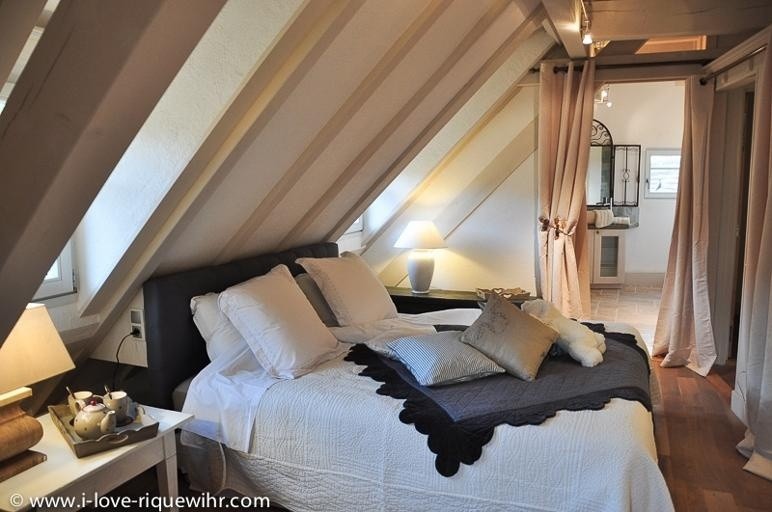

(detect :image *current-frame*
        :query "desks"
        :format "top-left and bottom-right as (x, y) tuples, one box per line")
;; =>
(0, 397), (195, 511)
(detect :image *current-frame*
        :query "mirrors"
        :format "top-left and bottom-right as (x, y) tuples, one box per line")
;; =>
(586, 145), (610, 207)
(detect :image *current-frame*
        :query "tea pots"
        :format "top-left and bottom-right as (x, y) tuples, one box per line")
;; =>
(72, 399), (117, 440)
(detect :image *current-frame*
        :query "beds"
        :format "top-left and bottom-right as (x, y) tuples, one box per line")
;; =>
(144, 242), (660, 510)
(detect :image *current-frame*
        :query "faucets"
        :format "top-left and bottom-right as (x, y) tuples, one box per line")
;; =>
(603, 197), (613, 211)
(596, 197), (607, 207)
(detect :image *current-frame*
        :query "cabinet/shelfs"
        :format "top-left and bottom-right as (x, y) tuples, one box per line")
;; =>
(584, 230), (625, 289)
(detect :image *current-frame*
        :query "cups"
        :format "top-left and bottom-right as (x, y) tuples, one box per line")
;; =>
(66, 391), (92, 420)
(102, 390), (128, 423)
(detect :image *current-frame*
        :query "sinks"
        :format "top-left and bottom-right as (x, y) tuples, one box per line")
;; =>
(586, 208), (610, 224)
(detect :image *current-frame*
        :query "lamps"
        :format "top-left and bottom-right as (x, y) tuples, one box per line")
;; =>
(1, 301), (76, 481)
(393, 221), (447, 294)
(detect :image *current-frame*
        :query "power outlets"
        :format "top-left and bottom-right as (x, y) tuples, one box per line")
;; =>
(129, 310), (145, 341)
